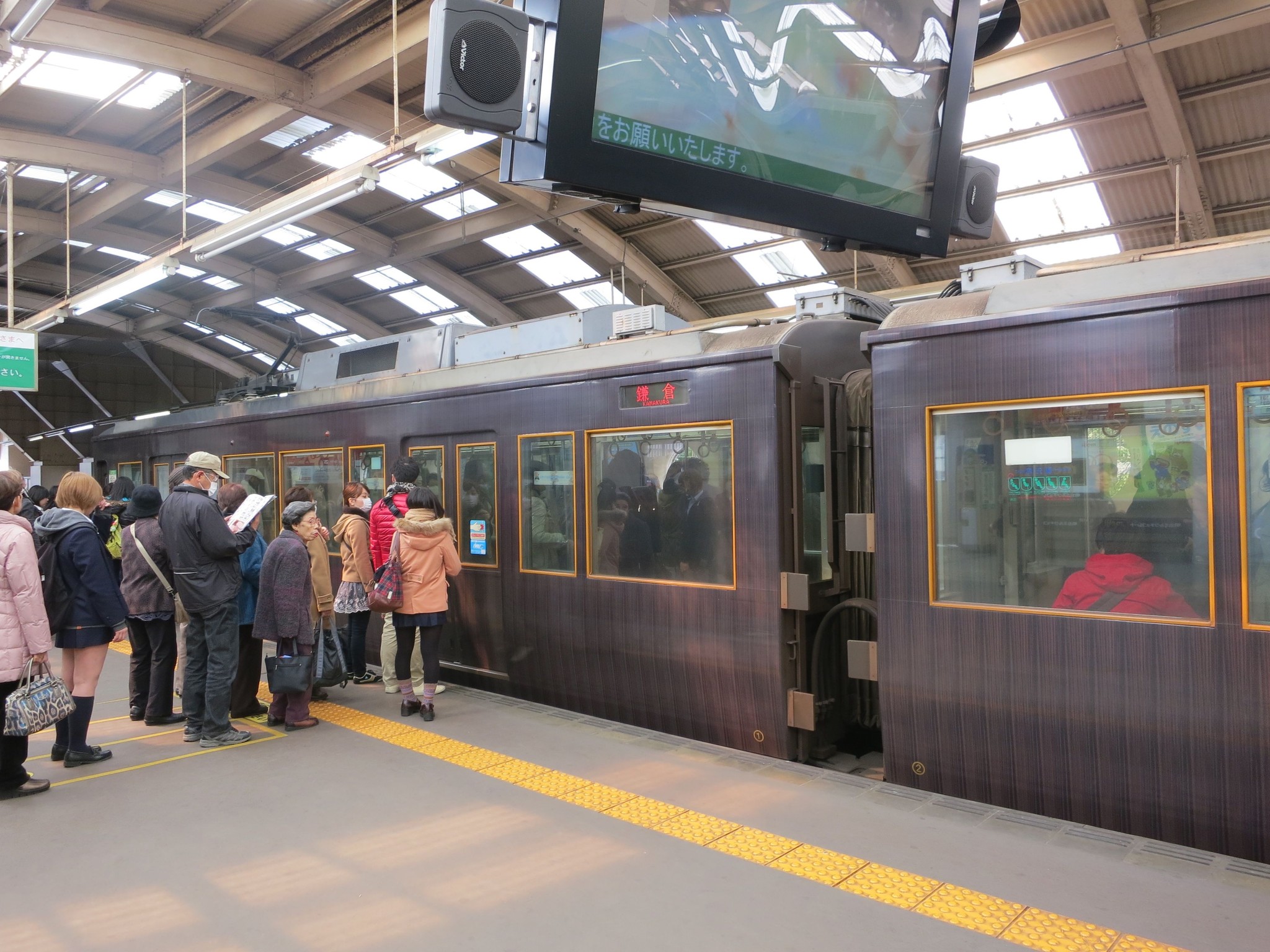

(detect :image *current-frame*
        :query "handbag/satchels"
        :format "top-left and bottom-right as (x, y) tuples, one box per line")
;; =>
(264, 636), (312, 695)
(3, 656), (76, 736)
(170, 591), (193, 627)
(365, 558), (403, 613)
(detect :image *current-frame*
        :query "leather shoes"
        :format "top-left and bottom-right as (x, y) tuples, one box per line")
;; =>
(50, 743), (112, 767)
(129, 705), (188, 726)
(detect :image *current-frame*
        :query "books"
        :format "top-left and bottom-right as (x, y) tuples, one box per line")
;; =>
(227, 494), (277, 533)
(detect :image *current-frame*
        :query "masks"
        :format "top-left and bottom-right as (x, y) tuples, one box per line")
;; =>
(353, 497), (372, 513)
(198, 471), (218, 497)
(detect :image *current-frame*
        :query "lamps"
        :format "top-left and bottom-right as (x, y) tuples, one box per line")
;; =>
(68, 256), (180, 316)
(190, 165), (379, 262)
(0, 0), (56, 45)
(25, 309), (68, 331)
(414, 124), (498, 166)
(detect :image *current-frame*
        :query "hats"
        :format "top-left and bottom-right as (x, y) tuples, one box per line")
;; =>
(126, 484), (163, 517)
(245, 468), (265, 479)
(184, 451), (230, 479)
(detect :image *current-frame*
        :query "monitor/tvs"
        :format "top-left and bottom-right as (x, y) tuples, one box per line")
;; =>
(498, 0), (978, 261)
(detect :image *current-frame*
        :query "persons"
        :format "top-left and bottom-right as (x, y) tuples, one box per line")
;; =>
(463, 449), (733, 583)
(463, 479), (490, 563)
(333, 456), (462, 721)
(0, 465), (52, 797)
(119, 449), (268, 747)
(1051, 512), (1203, 618)
(252, 486), (333, 731)
(90, 476), (135, 587)
(296, 469), (343, 552)
(1127, 442), (1207, 621)
(240, 469), (264, 537)
(1250, 459), (1270, 623)
(18, 485), (59, 551)
(34, 470), (129, 766)
(597, 485), (619, 577)
(414, 464), (443, 505)
(1063, 456), (1115, 583)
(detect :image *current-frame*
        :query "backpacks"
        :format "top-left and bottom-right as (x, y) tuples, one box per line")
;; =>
(105, 514), (123, 559)
(35, 520), (98, 636)
(310, 613), (348, 689)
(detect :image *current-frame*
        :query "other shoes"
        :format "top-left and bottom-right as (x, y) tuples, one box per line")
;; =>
(230, 704), (268, 718)
(0, 778), (51, 801)
(265, 712), (285, 726)
(310, 690), (328, 702)
(284, 717), (319, 732)
(401, 698), (435, 722)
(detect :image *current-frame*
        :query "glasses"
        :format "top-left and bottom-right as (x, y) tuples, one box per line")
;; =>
(300, 517), (320, 527)
(205, 472), (219, 482)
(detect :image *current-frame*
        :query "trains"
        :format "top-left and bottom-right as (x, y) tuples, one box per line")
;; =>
(89, 225), (1270, 864)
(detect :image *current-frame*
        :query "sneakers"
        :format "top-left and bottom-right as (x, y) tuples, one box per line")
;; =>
(200, 724), (252, 747)
(347, 669), (446, 696)
(183, 724), (202, 742)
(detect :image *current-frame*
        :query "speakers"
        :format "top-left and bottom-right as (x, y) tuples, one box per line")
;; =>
(423, 1), (530, 132)
(950, 154), (1000, 239)
